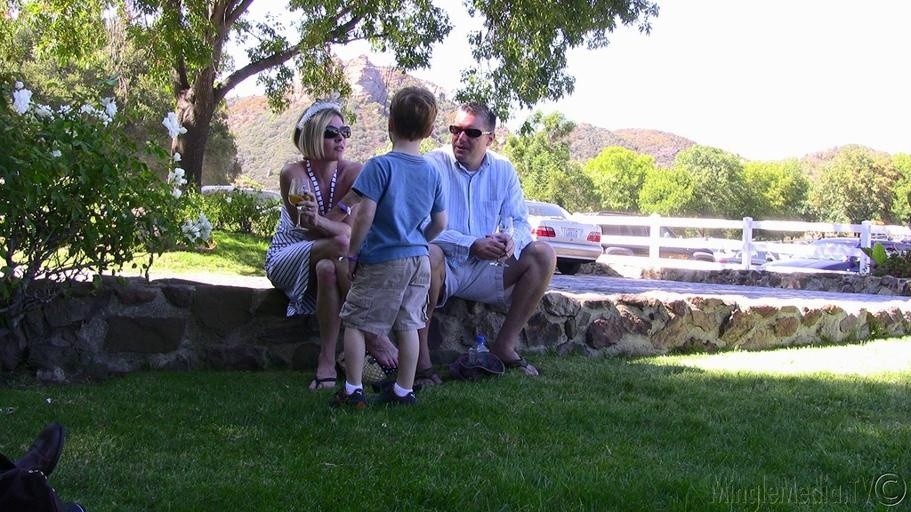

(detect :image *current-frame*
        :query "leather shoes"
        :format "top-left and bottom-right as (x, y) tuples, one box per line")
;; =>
(15, 421), (65, 476)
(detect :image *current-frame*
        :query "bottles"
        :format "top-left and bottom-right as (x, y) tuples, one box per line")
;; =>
(468, 335), (489, 362)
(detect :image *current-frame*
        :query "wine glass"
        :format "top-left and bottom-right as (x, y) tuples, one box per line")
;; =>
(287, 177), (311, 231)
(489, 215), (514, 267)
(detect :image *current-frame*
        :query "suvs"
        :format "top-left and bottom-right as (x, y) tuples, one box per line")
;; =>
(573, 212), (715, 261)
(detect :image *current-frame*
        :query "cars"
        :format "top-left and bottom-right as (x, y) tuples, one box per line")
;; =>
(703, 235), (910, 275)
(525, 204), (604, 275)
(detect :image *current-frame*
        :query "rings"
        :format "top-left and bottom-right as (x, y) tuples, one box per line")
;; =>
(308, 208), (311, 210)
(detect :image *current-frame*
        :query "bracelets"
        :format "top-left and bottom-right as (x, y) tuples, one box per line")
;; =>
(337, 201), (352, 215)
(347, 255), (358, 262)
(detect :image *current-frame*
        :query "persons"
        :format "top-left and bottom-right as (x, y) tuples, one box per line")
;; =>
(329, 86), (448, 408)
(264, 99), (400, 392)
(412, 103), (557, 390)
(0, 422), (86, 512)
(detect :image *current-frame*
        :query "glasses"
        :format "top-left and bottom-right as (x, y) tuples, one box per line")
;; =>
(326, 125), (352, 138)
(450, 125), (492, 138)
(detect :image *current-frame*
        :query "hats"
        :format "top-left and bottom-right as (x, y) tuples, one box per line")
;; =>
(448, 350), (506, 381)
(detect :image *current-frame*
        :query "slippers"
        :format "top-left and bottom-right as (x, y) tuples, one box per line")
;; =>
(503, 354), (530, 369)
(415, 367), (437, 380)
(310, 374), (338, 391)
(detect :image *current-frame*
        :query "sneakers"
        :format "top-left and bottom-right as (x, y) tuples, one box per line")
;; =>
(379, 383), (416, 405)
(332, 386), (368, 409)
